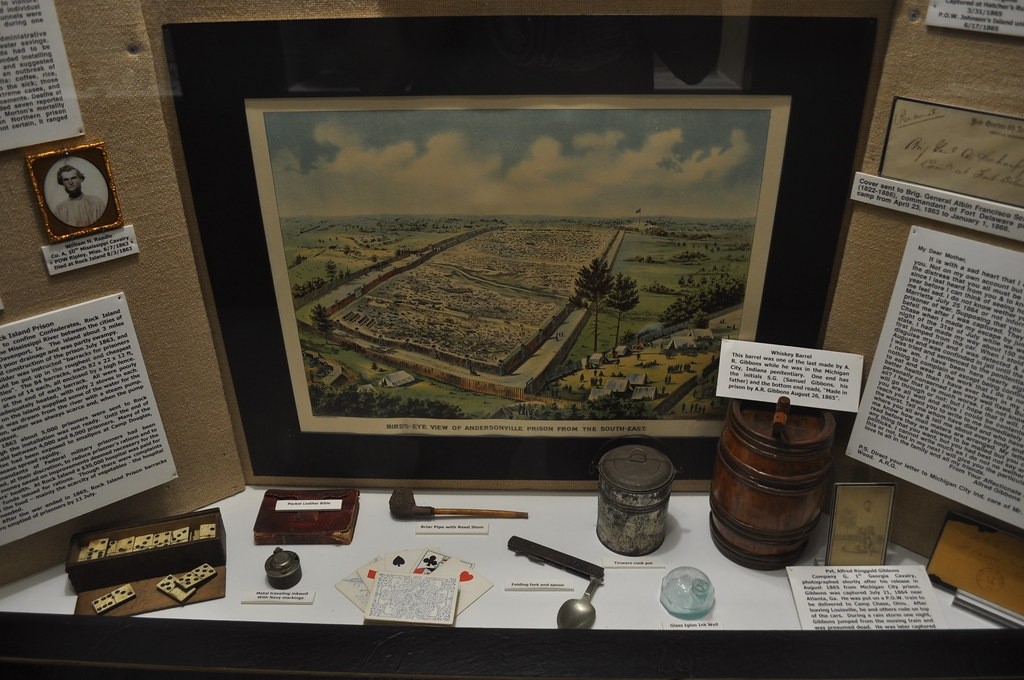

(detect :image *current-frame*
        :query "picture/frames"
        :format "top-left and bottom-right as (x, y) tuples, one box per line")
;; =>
(26, 140), (123, 242)
(824, 482), (897, 566)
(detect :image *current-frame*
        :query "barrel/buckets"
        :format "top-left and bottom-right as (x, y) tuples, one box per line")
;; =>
(593, 434), (676, 556)
(710, 397), (834, 570)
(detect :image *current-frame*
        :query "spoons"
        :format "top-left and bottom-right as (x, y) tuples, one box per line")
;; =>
(507, 536), (605, 627)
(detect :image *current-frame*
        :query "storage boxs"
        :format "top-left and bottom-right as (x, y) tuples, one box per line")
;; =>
(63, 507), (226, 592)
